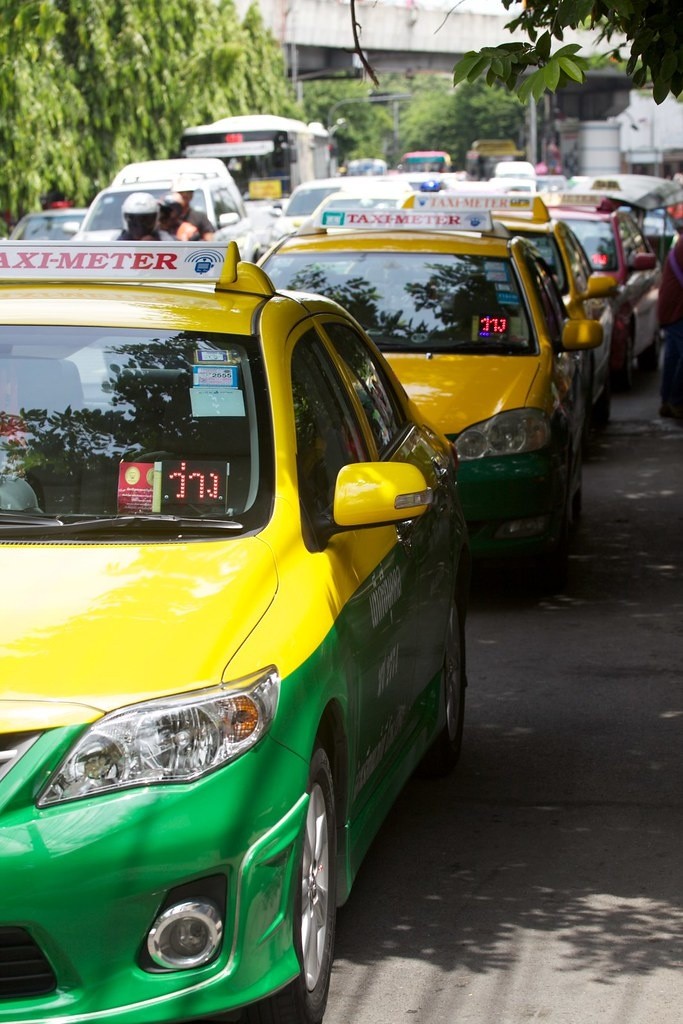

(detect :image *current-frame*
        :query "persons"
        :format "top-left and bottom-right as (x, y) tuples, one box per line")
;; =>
(119, 186), (215, 242)
(656, 222), (683, 424)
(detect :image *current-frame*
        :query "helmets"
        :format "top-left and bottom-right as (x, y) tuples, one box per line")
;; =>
(121, 192), (159, 232)
(157, 192), (186, 229)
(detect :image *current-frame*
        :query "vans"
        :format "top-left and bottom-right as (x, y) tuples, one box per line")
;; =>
(61, 157), (246, 247)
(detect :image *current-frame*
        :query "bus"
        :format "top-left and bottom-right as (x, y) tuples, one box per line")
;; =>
(176, 113), (333, 206)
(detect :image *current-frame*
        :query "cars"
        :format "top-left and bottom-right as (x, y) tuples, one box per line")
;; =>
(0, 239), (469, 1024)
(0, 208), (87, 242)
(212, 137), (683, 458)
(250, 206), (603, 601)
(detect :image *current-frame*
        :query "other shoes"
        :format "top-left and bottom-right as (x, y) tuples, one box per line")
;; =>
(659, 404), (683, 418)
